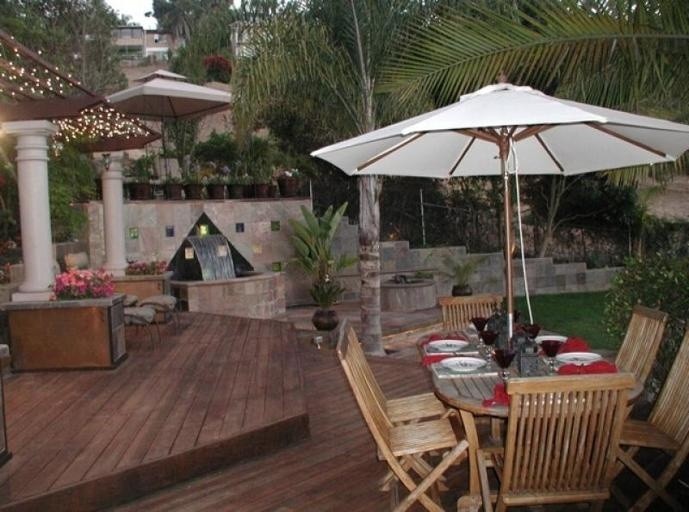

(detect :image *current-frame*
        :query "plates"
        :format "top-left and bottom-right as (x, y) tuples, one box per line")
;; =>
(535, 335), (602, 366)
(429, 339), (488, 372)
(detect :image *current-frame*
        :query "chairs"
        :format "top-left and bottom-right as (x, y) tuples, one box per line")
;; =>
(456, 371), (638, 511)
(611, 327), (689, 511)
(64, 250), (183, 333)
(437, 295), (505, 330)
(614, 303), (669, 421)
(336, 316), (457, 512)
(53, 257), (162, 346)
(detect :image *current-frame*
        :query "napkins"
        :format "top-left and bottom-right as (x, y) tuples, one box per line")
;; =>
(421, 354), (458, 367)
(418, 333), (466, 348)
(539, 334), (594, 354)
(482, 381), (513, 408)
(557, 360), (618, 375)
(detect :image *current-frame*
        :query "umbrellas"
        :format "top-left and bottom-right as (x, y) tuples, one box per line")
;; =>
(310, 72), (689, 353)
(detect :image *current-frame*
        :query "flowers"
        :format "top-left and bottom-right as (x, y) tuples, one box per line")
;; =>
(52, 267), (113, 299)
(125, 255), (166, 274)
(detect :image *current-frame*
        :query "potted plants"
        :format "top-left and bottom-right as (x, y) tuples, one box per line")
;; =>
(123, 155), (299, 201)
(419, 253), (492, 297)
(281, 201), (361, 330)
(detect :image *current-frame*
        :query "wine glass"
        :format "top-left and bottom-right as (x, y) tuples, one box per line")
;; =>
(470, 309), (564, 380)
